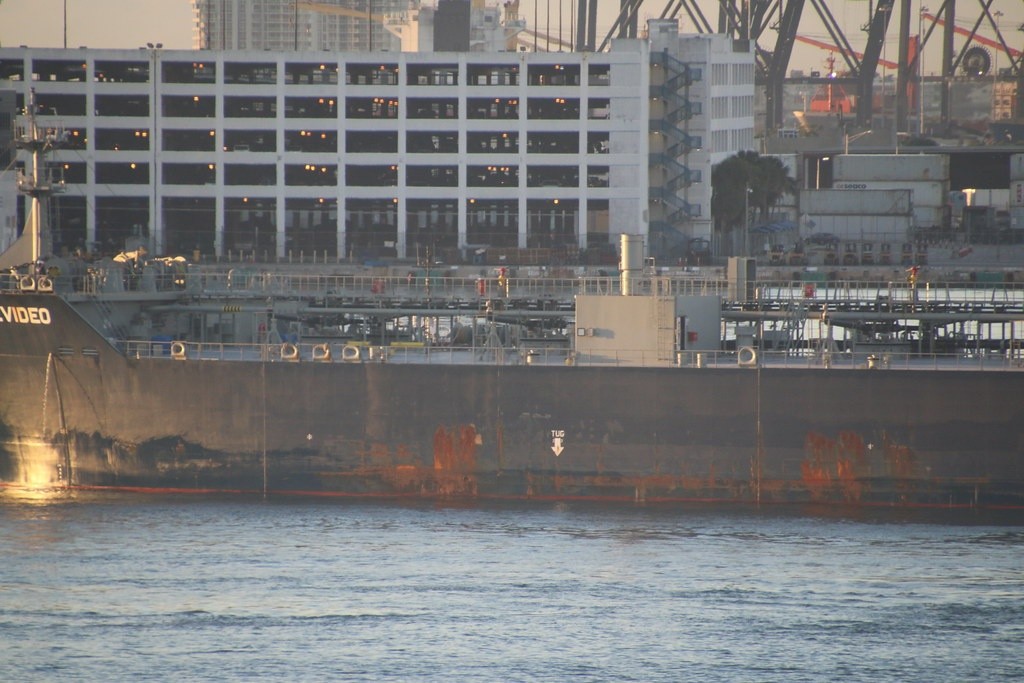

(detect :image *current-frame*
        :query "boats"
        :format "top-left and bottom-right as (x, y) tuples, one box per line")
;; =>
(0, 86), (1024, 522)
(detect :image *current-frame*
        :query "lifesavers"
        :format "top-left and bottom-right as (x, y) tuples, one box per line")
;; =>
(341, 345), (360, 360)
(38, 277), (55, 290)
(738, 346), (757, 365)
(20, 276), (36, 290)
(170, 342), (185, 357)
(312, 344), (331, 360)
(281, 345), (298, 359)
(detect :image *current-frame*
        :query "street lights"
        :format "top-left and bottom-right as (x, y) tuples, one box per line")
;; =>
(992, 10), (1004, 128)
(878, 5), (890, 133)
(919, 7), (931, 134)
(816, 154), (829, 190)
(846, 130), (873, 154)
(744, 186), (753, 259)
